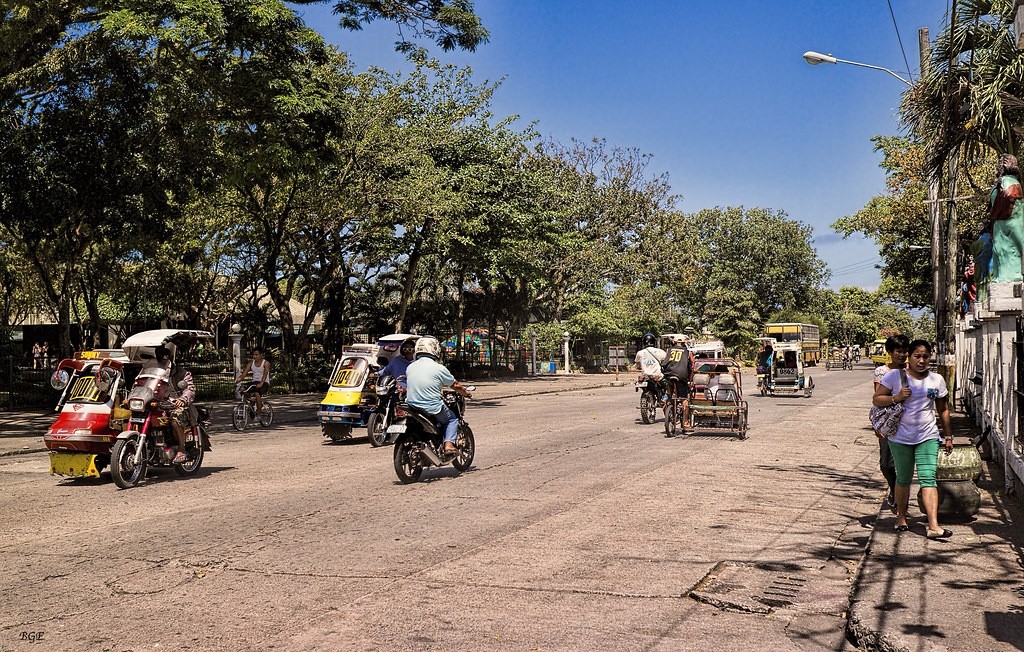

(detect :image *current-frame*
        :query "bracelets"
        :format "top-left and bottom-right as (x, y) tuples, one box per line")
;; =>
(892, 394), (896, 404)
(944, 435), (954, 440)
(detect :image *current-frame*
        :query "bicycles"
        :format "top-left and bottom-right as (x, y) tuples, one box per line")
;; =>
(232, 383), (274, 432)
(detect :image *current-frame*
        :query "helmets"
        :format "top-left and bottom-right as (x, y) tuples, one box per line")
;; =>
(415, 335), (441, 360)
(643, 334), (656, 346)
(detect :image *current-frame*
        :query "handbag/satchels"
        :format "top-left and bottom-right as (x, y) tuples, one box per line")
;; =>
(869, 369), (909, 437)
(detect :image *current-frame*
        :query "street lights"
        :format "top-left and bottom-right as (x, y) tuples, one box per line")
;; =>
(802, 52), (947, 378)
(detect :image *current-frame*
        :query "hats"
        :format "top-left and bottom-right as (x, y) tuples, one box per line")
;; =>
(674, 334), (685, 342)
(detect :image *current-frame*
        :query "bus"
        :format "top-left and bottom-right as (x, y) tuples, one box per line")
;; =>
(763, 323), (821, 367)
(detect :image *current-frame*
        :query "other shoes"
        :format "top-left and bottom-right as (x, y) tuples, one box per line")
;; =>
(253, 409), (261, 423)
(444, 449), (459, 455)
(888, 491), (896, 506)
(894, 517), (909, 531)
(927, 528), (953, 538)
(173, 453), (192, 462)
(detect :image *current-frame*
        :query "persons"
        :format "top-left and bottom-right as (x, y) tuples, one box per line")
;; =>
(690, 352), (708, 392)
(32, 342), (50, 369)
(370, 340), (415, 389)
(758, 345), (773, 368)
(155, 348), (195, 462)
(661, 334), (693, 427)
(635, 333), (666, 387)
(236, 348), (270, 422)
(845, 347), (859, 356)
(405, 335), (471, 454)
(874, 335), (910, 517)
(872, 340), (953, 539)
(704, 365), (737, 401)
(955, 153), (1024, 317)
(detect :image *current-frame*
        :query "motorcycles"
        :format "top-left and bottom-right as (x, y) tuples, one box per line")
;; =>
(386, 385), (476, 484)
(634, 375), (681, 425)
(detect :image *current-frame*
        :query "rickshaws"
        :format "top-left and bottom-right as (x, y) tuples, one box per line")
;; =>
(663, 357), (749, 440)
(755, 337), (815, 398)
(317, 333), (423, 448)
(825, 353), (853, 371)
(43, 329), (214, 488)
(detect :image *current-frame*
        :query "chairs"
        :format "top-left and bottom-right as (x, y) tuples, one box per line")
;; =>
(688, 387), (714, 426)
(715, 389), (741, 427)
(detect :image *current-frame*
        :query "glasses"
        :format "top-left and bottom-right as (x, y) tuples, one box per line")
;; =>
(403, 348), (415, 352)
